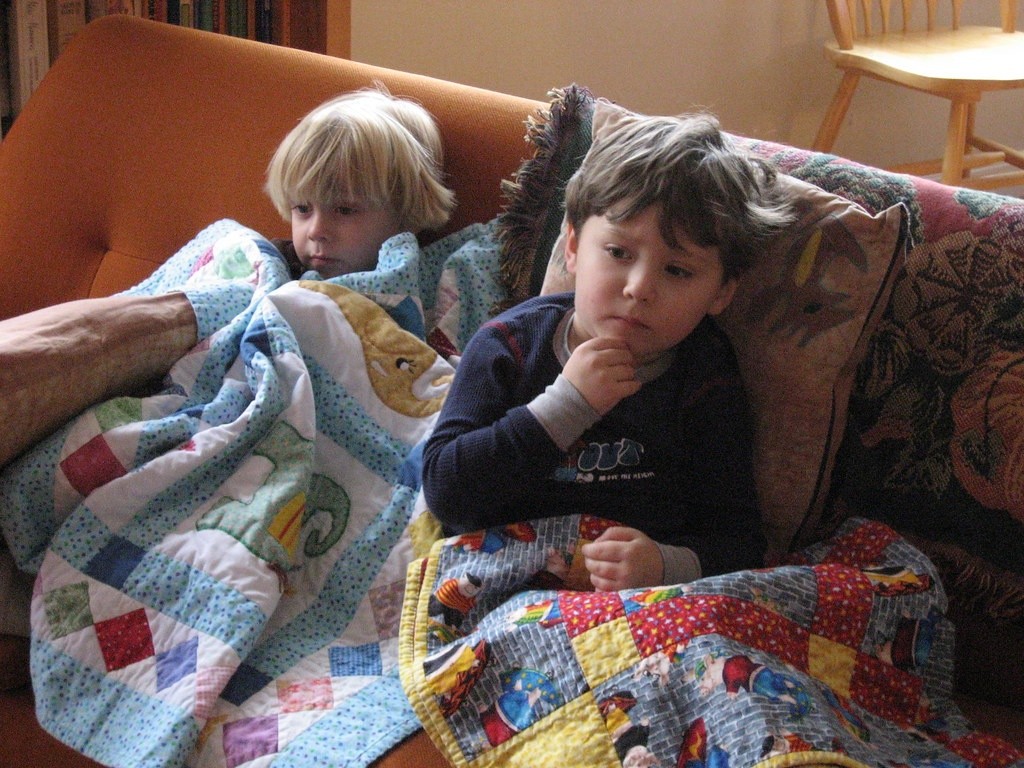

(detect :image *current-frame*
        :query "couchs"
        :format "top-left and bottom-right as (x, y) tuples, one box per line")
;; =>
(1, 11), (1024, 768)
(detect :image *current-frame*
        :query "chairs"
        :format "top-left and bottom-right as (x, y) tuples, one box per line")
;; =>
(810, 0), (1024, 190)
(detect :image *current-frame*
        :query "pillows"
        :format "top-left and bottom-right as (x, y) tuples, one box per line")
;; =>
(0, 290), (198, 469)
(541, 95), (910, 552)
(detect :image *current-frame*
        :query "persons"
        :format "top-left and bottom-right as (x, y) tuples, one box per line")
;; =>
(261, 86), (458, 283)
(420, 112), (768, 591)
